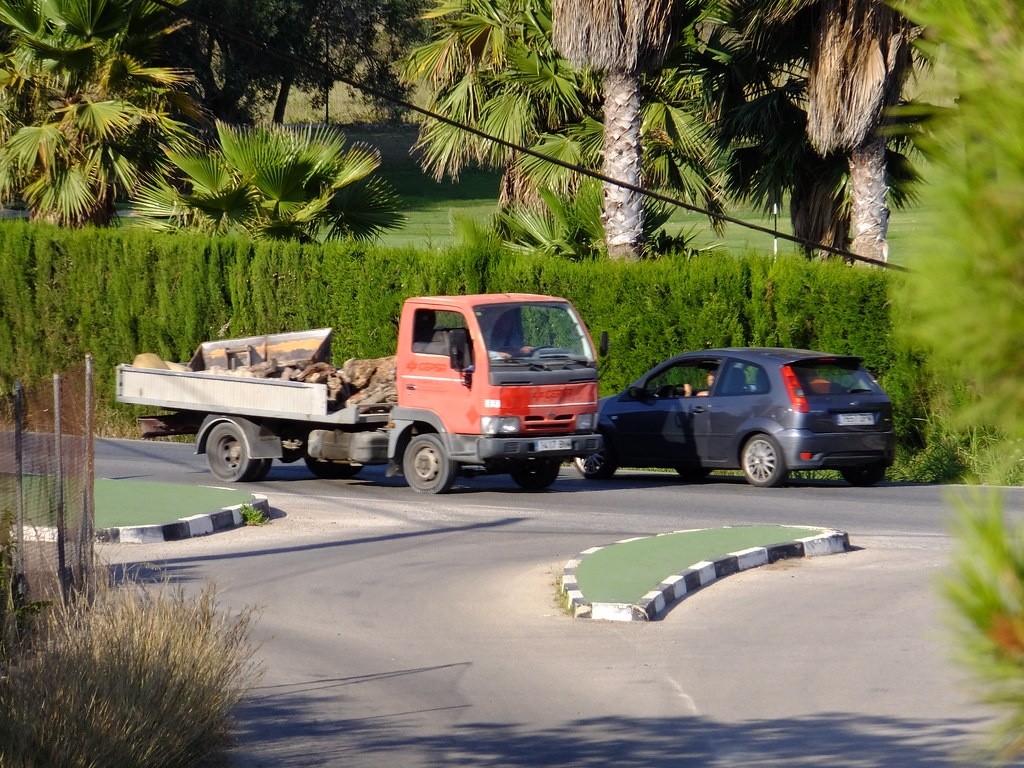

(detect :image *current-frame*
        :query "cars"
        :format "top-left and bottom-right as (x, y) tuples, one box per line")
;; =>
(572, 345), (897, 487)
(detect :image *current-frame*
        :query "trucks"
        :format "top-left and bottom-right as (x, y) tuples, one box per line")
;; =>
(114, 292), (610, 495)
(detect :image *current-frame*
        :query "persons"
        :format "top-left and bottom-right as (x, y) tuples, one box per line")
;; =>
(683, 372), (716, 397)
(484, 308), (534, 355)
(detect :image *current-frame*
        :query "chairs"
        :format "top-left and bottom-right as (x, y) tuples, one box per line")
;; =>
(440, 329), (470, 370)
